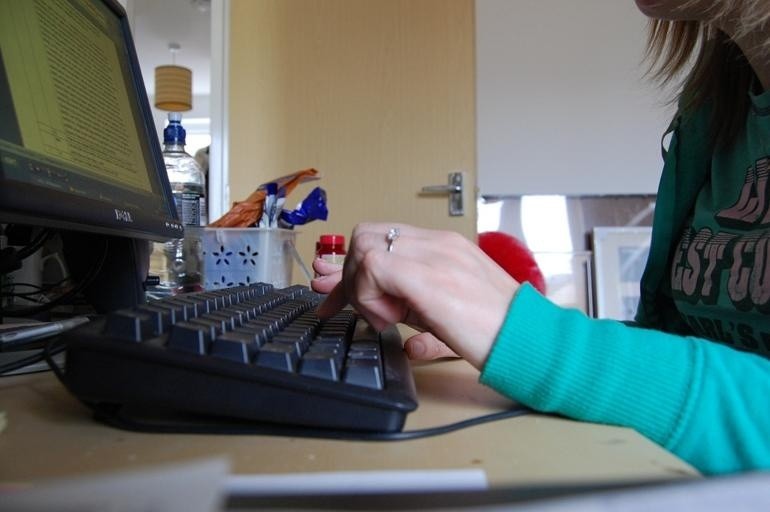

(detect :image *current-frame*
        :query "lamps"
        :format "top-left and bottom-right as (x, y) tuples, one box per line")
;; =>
(153, 42), (194, 112)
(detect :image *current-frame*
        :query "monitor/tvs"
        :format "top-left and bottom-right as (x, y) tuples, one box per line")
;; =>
(0, 0), (185, 344)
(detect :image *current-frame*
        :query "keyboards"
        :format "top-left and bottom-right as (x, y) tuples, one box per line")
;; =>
(62, 279), (419, 438)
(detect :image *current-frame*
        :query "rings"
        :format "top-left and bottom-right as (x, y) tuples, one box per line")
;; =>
(387, 228), (400, 252)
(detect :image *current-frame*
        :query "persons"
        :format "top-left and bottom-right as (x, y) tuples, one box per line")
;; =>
(311, 0), (770, 475)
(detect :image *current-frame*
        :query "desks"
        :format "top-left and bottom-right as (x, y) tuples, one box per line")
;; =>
(1, 327), (702, 484)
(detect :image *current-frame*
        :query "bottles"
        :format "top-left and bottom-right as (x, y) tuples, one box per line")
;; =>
(143, 110), (206, 303)
(317, 235), (347, 268)
